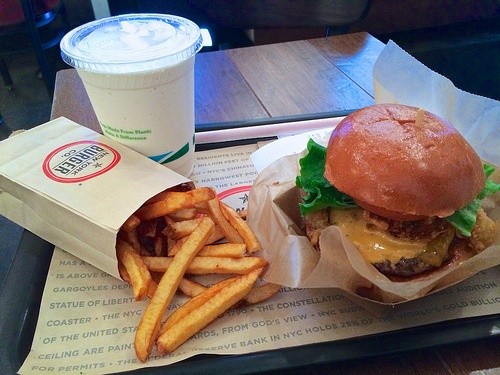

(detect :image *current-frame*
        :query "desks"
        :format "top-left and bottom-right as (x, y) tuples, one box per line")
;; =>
(49, 30), (500, 374)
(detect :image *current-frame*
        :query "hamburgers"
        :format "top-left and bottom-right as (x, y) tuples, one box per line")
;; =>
(273, 103), (500, 299)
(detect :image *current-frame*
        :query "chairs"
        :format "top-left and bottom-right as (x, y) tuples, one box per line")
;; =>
(200, 0), (372, 45)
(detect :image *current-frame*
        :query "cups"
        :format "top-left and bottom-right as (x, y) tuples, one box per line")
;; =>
(58, 13), (205, 179)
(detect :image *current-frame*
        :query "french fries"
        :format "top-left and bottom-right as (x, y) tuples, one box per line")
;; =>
(117, 186), (281, 364)
